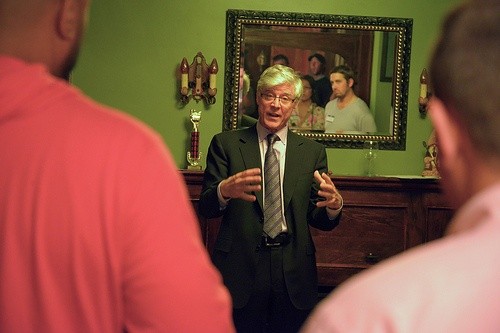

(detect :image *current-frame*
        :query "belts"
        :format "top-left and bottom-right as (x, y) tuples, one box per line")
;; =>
(258, 237), (287, 248)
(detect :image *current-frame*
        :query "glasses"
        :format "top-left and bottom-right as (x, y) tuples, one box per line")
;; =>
(260, 93), (297, 105)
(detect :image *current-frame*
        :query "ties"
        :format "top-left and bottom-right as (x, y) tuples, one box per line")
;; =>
(264, 133), (284, 238)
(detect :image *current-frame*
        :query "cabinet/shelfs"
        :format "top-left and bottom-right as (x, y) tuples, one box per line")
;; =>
(179, 170), (455, 300)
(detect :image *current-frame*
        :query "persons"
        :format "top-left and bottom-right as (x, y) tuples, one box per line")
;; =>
(0, 0), (234, 333)
(299, 0), (500, 333)
(238, 53), (378, 136)
(198, 64), (344, 333)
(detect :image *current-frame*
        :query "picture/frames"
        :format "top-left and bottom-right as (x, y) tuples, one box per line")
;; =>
(222, 9), (414, 151)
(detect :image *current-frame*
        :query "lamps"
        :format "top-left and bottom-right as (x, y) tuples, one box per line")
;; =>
(419, 69), (430, 114)
(180, 51), (218, 105)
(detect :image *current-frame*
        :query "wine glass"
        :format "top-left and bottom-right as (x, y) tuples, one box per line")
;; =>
(361, 140), (378, 176)
